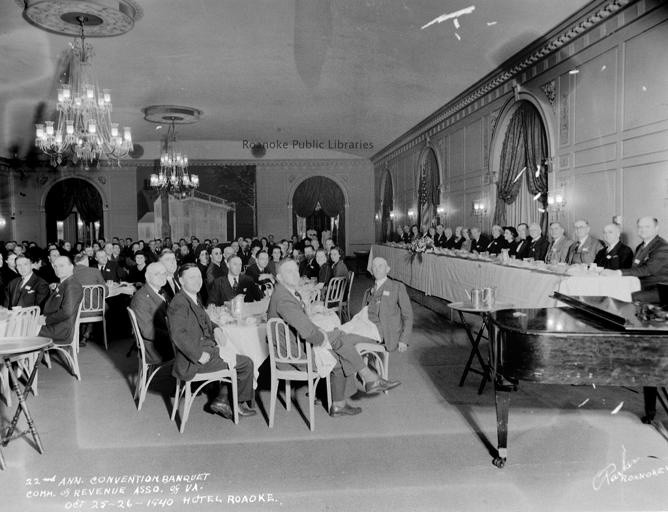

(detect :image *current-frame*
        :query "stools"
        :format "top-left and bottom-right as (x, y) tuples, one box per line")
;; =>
(353, 344), (390, 389)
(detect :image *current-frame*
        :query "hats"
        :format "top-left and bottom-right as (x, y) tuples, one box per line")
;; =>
(195, 243), (212, 258)
(502, 226), (518, 237)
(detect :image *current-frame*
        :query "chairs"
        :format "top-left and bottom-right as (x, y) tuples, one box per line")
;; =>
(321, 276), (347, 318)
(342, 271), (354, 319)
(1, 278), (137, 401)
(160, 315), (236, 432)
(265, 316), (332, 430)
(126, 308), (165, 409)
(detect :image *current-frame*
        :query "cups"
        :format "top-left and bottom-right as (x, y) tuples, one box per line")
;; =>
(208, 276), (318, 326)
(484, 289), (495, 306)
(387, 241), (605, 277)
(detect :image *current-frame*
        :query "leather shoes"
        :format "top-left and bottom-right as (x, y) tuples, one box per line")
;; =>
(210, 399), (233, 418)
(238, 401), (256, 416)
(366, 376), (401, 394)
(331, 403), (362, 417)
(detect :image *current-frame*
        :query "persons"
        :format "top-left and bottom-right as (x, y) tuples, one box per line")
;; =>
(0, 215), (668, 420)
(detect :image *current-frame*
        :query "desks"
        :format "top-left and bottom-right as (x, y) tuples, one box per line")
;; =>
(366, 241), (637, 336)
(1, 337), (53, 467)
(446, 299), (512, 395)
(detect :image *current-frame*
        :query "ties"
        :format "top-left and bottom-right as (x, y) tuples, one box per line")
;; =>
(233, 278), (238, 289)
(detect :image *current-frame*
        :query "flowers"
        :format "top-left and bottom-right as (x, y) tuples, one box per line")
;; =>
(405, 232), (436, 264)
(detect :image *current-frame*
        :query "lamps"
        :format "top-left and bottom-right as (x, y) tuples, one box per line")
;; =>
(137, 103), (206, 201)
(370, 192), (568, 221)
(24, 0), (141, 174)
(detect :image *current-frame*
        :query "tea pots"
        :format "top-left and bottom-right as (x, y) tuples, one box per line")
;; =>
(463, 288), (491, 307)
